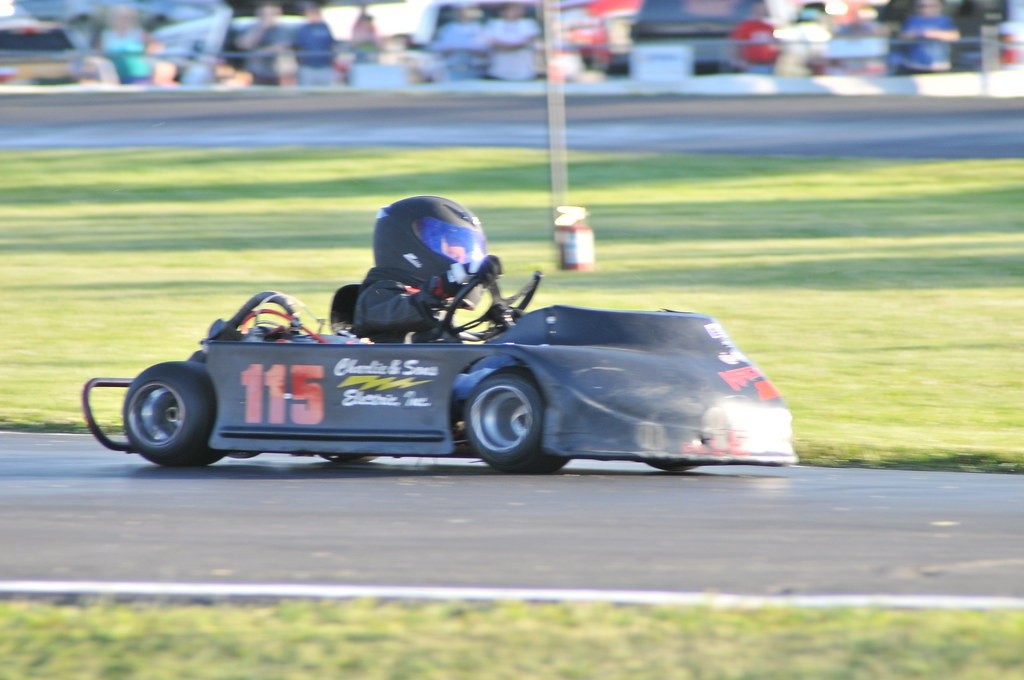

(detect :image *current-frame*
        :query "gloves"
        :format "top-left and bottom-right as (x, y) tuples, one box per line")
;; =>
(437, 256), (503, 293)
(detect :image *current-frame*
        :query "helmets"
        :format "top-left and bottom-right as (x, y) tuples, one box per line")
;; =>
(374, 196), (485, 297)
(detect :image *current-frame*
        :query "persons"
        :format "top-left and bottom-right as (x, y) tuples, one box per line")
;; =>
(431, 3), (484, 82)
(213, 0), (382, 90)
(734, 1), (961, 75)
(485, 3), (541, 80)
(351, 195), (504, 345)
(96, 3), (178, 85)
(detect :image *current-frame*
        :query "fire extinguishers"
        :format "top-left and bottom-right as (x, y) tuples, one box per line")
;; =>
(552, 205), (595, 270)
